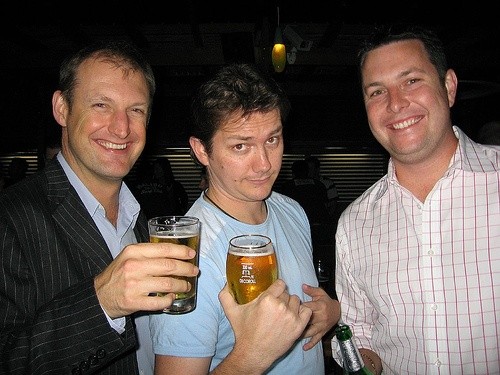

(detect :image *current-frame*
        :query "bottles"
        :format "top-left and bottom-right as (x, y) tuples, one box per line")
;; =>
(334, 324), (376, 375)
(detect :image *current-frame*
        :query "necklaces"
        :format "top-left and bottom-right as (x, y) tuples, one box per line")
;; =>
(203, 187), (268, 224)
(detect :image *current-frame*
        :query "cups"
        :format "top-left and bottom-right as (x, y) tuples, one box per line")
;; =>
(225, 234), (279, 305)
(147, 216), (201, 315)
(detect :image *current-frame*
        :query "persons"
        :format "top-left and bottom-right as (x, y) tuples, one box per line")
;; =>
(6, 157), (28, 189)
(0, 39), (199, 375)
(331, 25), (500, 375)
(149, 63), (341, 375)
(281, 153), (339, 223)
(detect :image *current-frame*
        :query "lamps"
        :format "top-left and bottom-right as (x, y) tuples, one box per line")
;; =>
(272, 6), (286, 74)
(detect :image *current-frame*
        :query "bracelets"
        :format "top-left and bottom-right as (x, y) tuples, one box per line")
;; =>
(360, 353), (378, 374)
